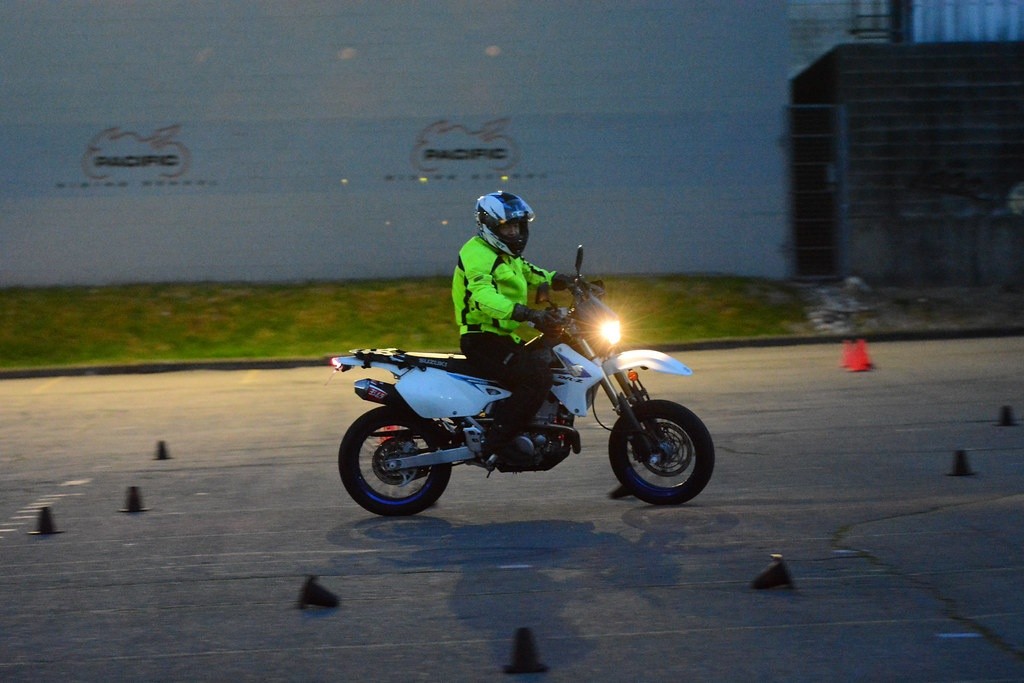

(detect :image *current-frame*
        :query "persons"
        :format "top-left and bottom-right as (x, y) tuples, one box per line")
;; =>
(451, 191), (605, 464)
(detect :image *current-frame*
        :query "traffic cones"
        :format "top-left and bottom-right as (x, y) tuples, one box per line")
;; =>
(837, 336), (873, 373)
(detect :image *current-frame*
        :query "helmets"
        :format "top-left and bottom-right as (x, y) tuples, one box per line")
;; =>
(475, 191), (535, 258)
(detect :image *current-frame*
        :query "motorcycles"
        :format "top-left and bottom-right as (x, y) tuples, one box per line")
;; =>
(329, 242), (717, 519)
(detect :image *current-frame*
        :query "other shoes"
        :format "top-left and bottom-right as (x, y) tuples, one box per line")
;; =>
(480, 432), (530, 468)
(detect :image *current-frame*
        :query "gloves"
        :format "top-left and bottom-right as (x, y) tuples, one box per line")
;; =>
(528, 308), (557, 326)
(553, 273), (581, 295)
